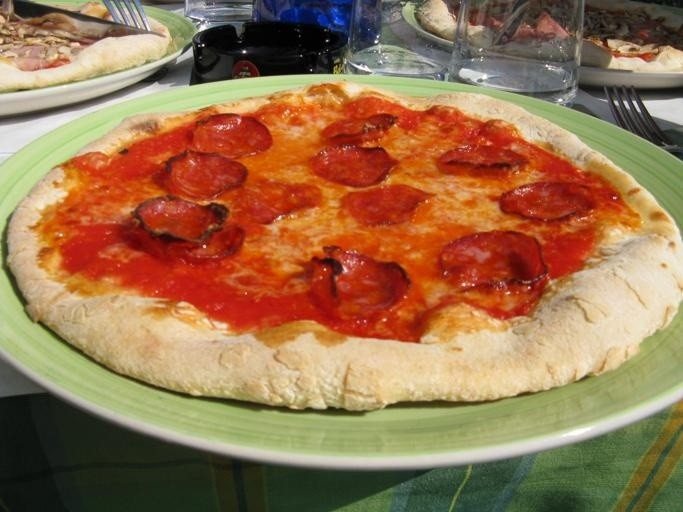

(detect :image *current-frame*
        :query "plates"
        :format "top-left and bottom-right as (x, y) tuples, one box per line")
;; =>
(1, 1), (196, 116)
(3, 72), (683, 473)
(395, 1), (683, 93)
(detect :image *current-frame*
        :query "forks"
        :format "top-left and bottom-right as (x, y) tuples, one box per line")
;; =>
(104, 1), (151, 31)
(604, 83), (683, 159)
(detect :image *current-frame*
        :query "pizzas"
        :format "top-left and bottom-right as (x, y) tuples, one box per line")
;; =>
(6, 83), (683, 410)
(1, 0), (172, 96)
(414, 0), (682, 77)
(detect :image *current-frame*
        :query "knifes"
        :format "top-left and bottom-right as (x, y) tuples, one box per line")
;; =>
(0, 0), (168, 40)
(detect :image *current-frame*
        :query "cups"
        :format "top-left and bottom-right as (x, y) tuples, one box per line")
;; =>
(344, 1), (462, 78)
(180, 1), (252, 28)
(448, 0), (586, 107)
